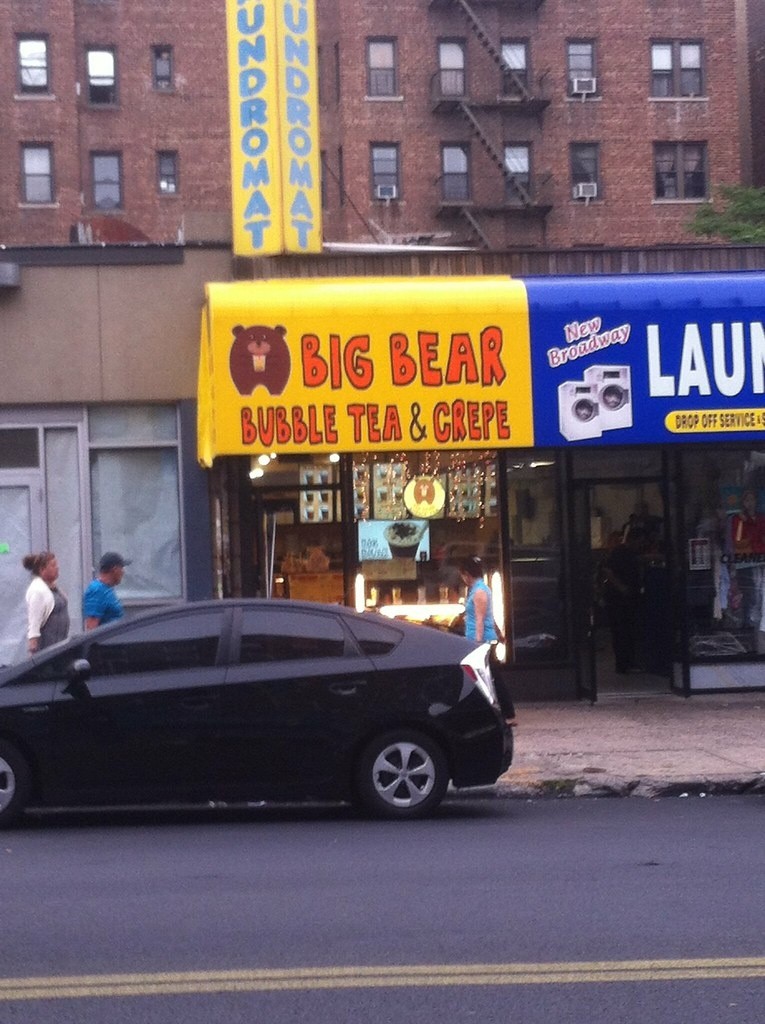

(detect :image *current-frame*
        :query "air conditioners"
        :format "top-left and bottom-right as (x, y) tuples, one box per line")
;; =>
(574, 182), (598, 200)
(569, 77), (597, 97)
(377, 183), (397, 201)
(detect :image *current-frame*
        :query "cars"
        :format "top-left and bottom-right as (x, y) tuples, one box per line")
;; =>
(1, 599), (514, 824)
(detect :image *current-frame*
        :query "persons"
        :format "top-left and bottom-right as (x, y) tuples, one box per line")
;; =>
(603, 498), (666, 673)
(455, 557), (519, 727)
(22, 550), (70, 653)
(81, 552), (134, 632)
(277, 534), (330, 599)
(688, 465), (765, 629)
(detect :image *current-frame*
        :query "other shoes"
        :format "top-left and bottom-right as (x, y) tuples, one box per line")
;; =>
(615, 662), (646, 676)
(505, 718), (518, 726)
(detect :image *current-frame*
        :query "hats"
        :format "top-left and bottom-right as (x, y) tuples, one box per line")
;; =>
(98, 552), (131, 570)
